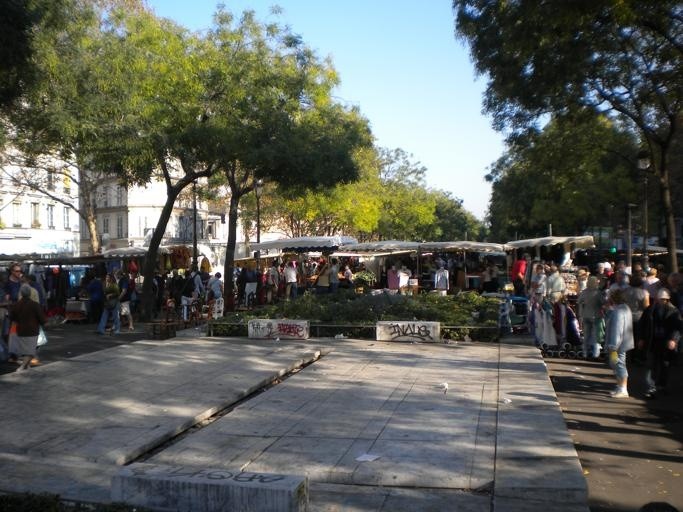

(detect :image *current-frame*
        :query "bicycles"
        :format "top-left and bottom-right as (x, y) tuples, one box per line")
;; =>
(164, 296), (204, 332)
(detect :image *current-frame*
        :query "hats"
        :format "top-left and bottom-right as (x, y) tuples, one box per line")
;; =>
(657, 288), (671, 299)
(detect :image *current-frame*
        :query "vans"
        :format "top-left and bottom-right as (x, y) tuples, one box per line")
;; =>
(55, 265), (87, 306)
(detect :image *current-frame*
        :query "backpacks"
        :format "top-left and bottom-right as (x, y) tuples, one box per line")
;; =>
(187, 277), (196, 291)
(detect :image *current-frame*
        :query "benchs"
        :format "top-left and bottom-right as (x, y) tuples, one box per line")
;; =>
(145, 301), (213, 341)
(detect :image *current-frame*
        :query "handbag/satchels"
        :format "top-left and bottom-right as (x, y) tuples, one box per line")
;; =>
(104, 300), (117, 309)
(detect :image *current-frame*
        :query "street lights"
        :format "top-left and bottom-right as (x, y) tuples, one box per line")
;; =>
(634, 147), (653, 271)
(253, 177), (264, 277)
(191, 170), (198, 274)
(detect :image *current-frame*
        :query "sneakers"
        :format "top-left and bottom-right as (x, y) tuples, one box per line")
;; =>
(608, 390), (629, 398)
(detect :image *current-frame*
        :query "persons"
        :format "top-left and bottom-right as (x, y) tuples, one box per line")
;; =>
(1, 253), (223, 373)
(237, 255), (353, 306)
(381, 254), (528, 315)
(531, 254), (683, 399)
